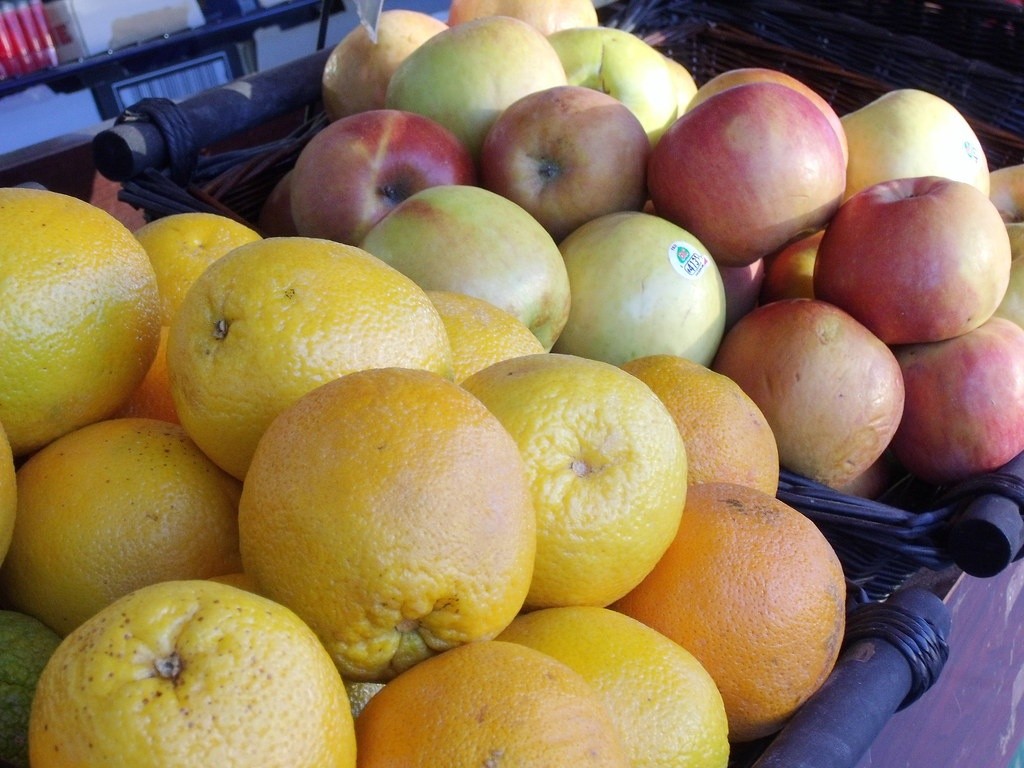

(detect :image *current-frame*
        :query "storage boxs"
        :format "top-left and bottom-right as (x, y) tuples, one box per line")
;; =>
(0, 0), (1024, 768)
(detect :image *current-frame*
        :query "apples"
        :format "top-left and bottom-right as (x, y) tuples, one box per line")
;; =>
(267, 0), (1024, 512)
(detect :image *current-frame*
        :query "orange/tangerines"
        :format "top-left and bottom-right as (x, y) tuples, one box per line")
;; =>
(0, 186), (848, 768)
